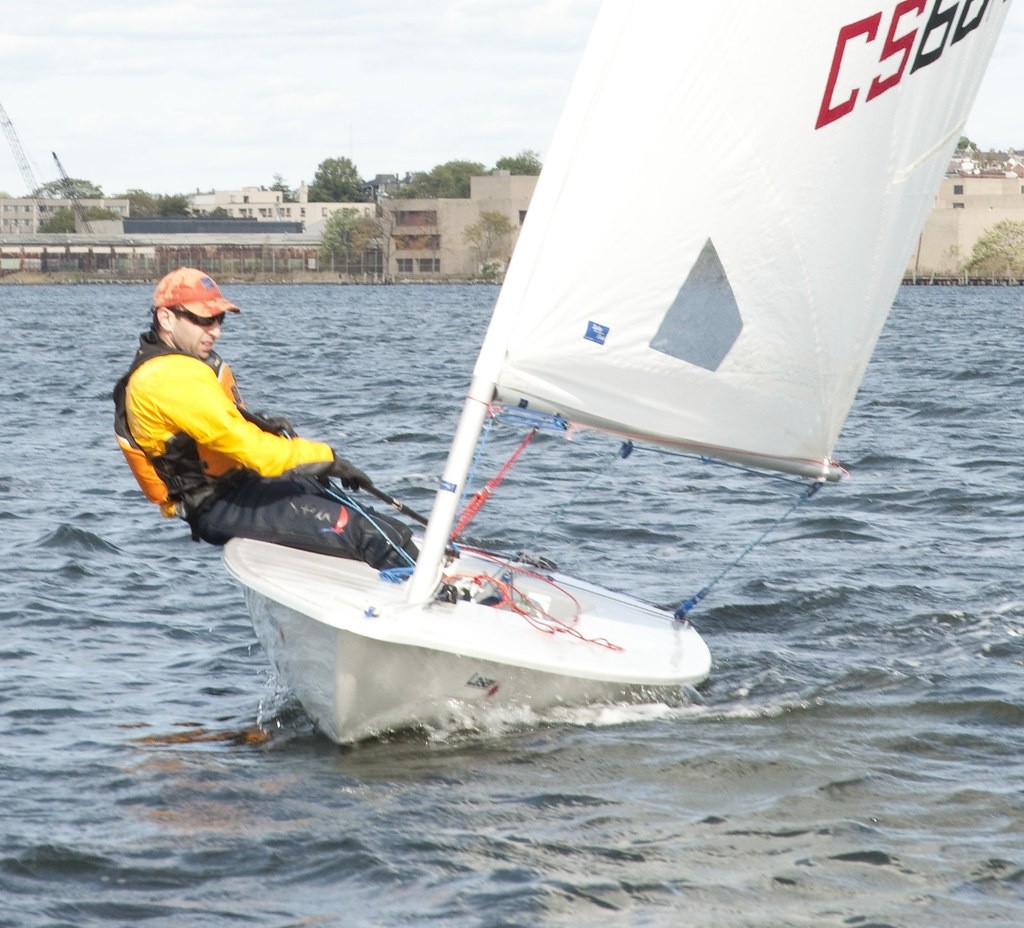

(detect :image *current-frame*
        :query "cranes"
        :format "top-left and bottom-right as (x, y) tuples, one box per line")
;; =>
(51, 151), (96, 236)
(0, 104), (56, 236)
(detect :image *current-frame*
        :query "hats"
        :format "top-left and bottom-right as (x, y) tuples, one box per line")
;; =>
(152, 265), (241, 318)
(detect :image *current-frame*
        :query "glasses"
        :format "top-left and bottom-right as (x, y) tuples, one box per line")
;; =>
(171, 308), (225, 326)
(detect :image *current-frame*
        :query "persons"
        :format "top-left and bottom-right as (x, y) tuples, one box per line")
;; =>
(115, 265), (476, 601)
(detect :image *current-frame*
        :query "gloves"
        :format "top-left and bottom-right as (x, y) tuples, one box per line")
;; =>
(330, 450), (374, 493)
(268, 416), (298, 439)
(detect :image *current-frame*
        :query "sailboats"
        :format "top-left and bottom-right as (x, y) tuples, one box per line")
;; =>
(210, 0), (1010, 759)
